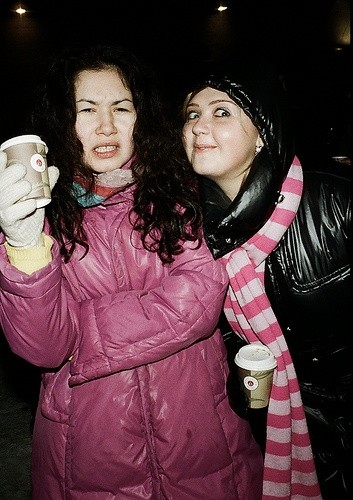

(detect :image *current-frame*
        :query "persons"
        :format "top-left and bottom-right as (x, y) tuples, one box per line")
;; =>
(177, 62), (353, 500)
(0, 39), (265, 499)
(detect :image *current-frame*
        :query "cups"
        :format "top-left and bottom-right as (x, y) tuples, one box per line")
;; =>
(1, 135), (51, 209)
(235, 344), (277, 409)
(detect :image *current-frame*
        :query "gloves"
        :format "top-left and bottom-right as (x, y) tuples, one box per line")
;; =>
(1, 153), (61, 248)
